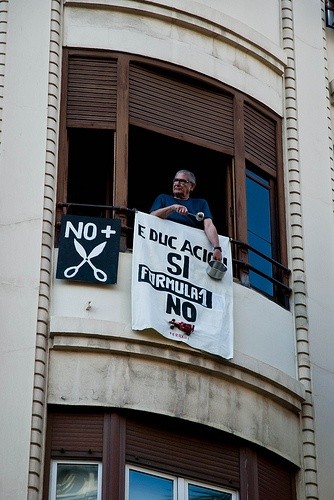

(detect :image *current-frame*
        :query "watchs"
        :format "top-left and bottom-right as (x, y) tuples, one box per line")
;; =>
(213, 246), (222, 252)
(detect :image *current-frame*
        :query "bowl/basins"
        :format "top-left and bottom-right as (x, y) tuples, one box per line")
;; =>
(206, 260), (227, 279)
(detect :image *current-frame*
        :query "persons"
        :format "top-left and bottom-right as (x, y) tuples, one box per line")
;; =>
(149, 169), (223, 262)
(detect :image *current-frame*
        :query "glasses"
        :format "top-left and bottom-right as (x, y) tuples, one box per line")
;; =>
(173, 179), (191, 185)
